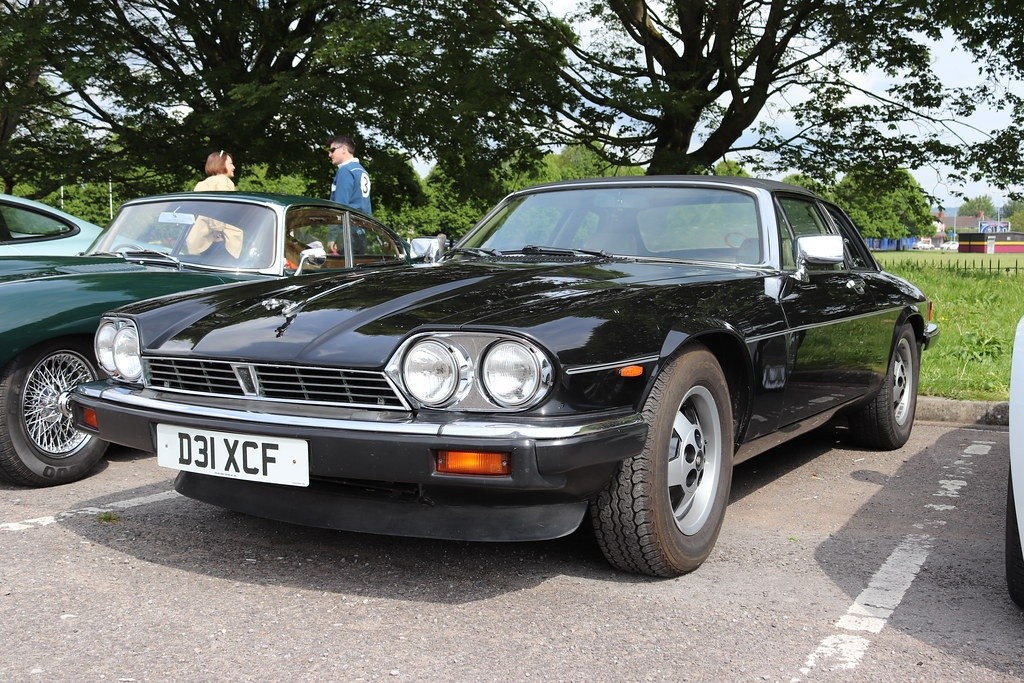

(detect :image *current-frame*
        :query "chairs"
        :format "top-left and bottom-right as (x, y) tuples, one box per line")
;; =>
(586, 228), (635, 255)
(738, 238), (794, 268)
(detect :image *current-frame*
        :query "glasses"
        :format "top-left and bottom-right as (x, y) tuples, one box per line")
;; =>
(328, 146), (343, 153)
(219, 150), (227, 158)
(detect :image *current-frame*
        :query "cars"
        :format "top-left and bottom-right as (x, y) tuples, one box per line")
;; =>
(940, 241), (959, 251)
(63, 172), (943, 578)
(0, 192), (416, 490)
(0, 189), (183, 257)
(912, 240), (935, 251)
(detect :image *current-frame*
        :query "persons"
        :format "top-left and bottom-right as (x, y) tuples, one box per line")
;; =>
(327, 135), (372, 255)
(185, 150), (243, 268)
